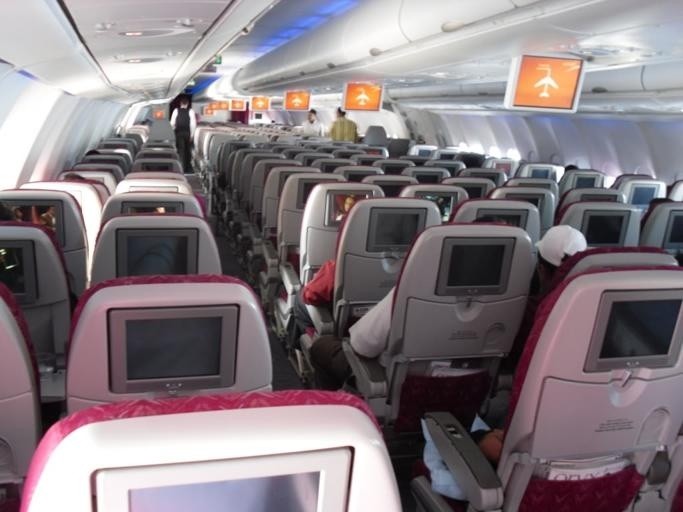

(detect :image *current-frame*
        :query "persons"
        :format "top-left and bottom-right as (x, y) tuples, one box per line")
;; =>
(437, 197), (446, 220)
(301, 108), (326, 139)
(13, 207), (23, 222)
(309, 282), (397, 391)
(471, 414), (511, 469)
(298, 258), (337, 334)
(330, 107), (358, 143)
(38, 206), (54, 232)
(509, 223), (590, 365)
(333, 196), (355, 224)
(169, 98), (196, 172)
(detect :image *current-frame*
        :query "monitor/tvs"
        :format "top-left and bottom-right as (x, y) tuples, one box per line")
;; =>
(434, 238), (515, 296)
(108, 305), (238, 394)
(0, 158), (198, 306)
(341, 80), (384, 112)
(153, 89), (312, 120)
(95, 446), (351, 512)
(583, 289), (683, 372)
(367, 208), (426, 252)
(581, 210), (631, 247)
(662, 211), (683, 252)
(503, 48), (588, 114)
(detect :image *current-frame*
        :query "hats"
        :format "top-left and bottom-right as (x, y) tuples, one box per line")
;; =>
(535, 225), (587, 267)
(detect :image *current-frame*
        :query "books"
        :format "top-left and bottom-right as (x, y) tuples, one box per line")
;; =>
(532, 454), (633, 483)
